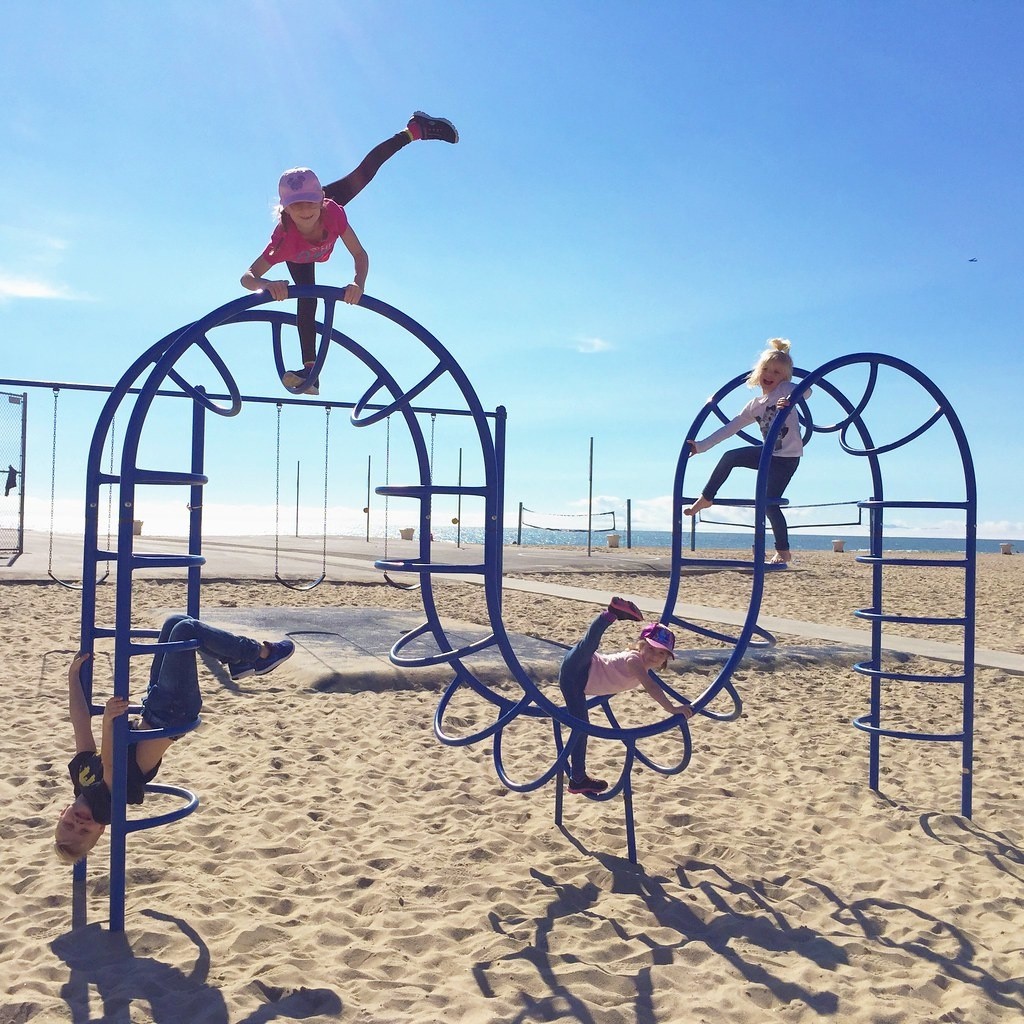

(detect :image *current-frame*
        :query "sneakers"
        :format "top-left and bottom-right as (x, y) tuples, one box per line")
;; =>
(282, 366), (319, 395)
(407, 110), (459, 144)
(254, 639), (296, 676)
(567, 775), (608, 794)
(228, 661), (255, 680)
(608, 597), (643, 622)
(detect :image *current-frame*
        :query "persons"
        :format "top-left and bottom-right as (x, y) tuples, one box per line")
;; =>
(55, 615), (294, 863)
(559, 597), (695, 794)
(241, 112), (459, 395)
(684, 340), (812, 563)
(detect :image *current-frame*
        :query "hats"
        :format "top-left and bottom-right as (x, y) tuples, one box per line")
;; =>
(639, 622), (675, 661)
(278, 166), (323, 213)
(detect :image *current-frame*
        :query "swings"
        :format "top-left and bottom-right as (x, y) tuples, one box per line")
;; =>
(269, 406), (334, 592)
(382, 417), (440, 592)
(45, 391), (116, 594)
